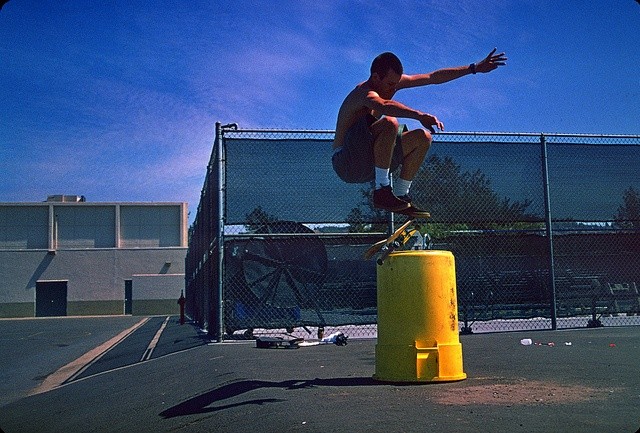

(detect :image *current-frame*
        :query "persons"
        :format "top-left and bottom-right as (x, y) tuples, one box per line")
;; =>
(331, 45), (507, 219)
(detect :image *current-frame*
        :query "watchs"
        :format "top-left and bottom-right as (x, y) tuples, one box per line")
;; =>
(468, 62), (478, 75)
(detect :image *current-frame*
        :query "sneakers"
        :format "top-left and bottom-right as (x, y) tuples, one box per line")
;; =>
(395, 193), (431, 219)
(373, 184), (411, 211)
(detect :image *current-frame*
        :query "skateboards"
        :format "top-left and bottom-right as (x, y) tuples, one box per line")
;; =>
(362, 217), (421, 265)
